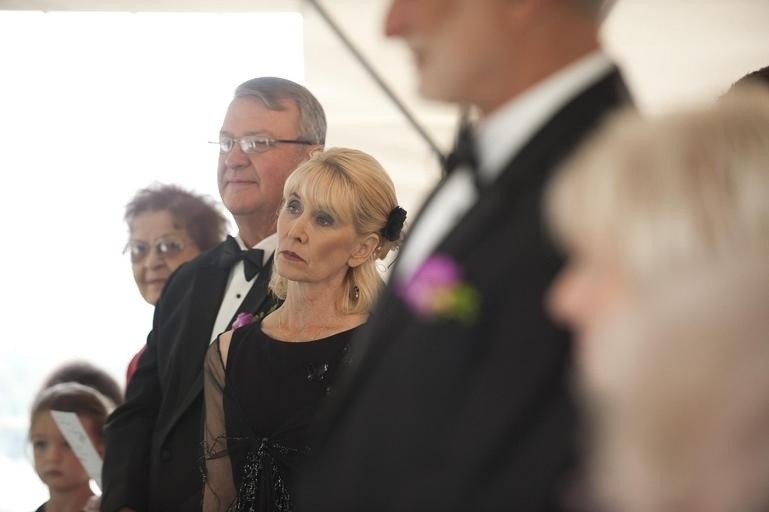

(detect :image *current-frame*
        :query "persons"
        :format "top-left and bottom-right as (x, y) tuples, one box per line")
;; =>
(540, 93), (769, 510)
(27, 383), (111, 511)
(303, 0), (639, 512)
(117, 179), (225, 403)
(202, 145), (409, 511)
(28, 364), (123, 413)
(102, 76), (335, 510)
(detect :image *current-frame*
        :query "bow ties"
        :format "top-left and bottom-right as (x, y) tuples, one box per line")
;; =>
(219, 235), (264, 282)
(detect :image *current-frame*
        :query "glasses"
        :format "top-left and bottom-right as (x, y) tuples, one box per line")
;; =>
(218, 135), (313, 154)
(130, 237), (194, 263)
